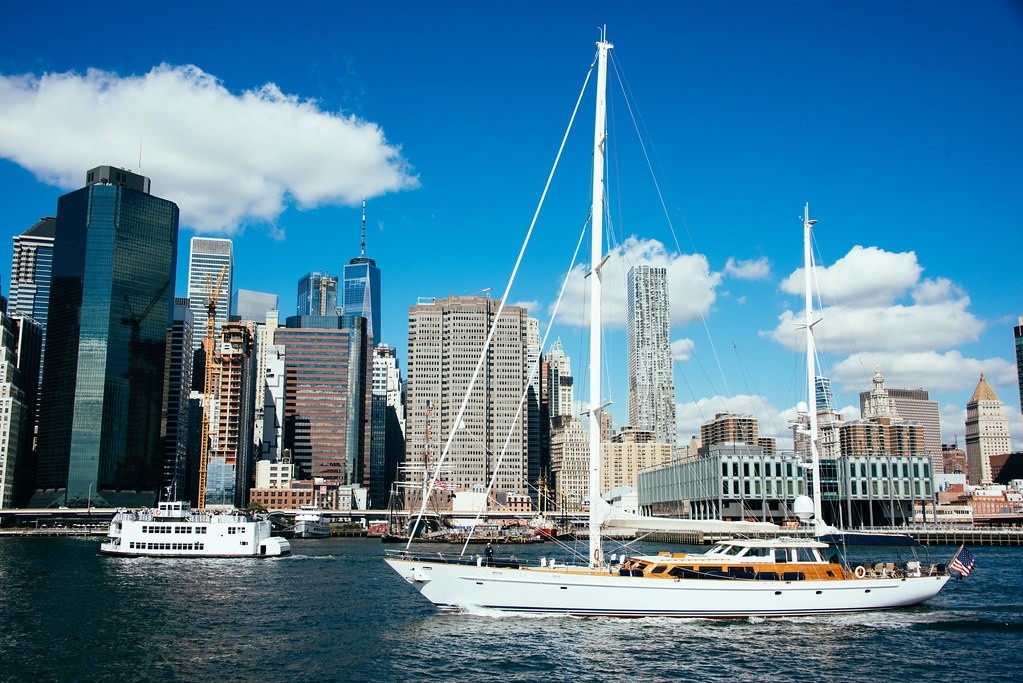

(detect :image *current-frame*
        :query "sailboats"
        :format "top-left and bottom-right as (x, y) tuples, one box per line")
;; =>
(394, 408), (459, 534)
(378, 22), (950, 617)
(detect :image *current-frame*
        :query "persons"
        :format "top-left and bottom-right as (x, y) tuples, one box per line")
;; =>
(899, 564), (905, 578)
(892, 563), (899, 578)
(128, 509), (150, 521)
(485, 542), (494, 566)
(829, 553), (838, 563)
(192, 509), (242, 515)
(775, 548), (789, 564)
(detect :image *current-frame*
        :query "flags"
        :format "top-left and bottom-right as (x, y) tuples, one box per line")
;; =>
(950, 547), (975, 576)
(433, 481), (448, 492)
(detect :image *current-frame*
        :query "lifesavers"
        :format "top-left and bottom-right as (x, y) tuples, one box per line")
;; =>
(855, 565), (866, 579)
(594, 548), (600, 561)
(156, 510), (161, 515)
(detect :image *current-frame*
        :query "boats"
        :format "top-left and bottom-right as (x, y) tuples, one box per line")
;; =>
(292, 505), (329, 539)
(97, 501), (289, 559)
